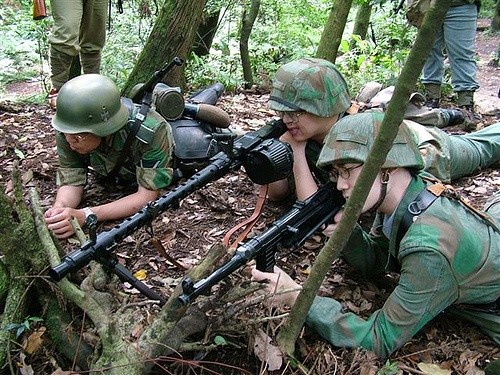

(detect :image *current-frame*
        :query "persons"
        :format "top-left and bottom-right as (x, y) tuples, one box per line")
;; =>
(267, 59), (500, 204)
(51, 0), (108, 109)
(43, 75), (239, 239)
(408, 0), (479, 114)
(246, 111), (500, 359)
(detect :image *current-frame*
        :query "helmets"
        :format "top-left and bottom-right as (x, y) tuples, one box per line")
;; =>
(51, 73), (130, 137)
(315, 109), (425, 173)
(265, 58), (352, 118)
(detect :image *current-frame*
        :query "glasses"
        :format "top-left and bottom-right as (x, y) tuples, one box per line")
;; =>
(274, 110), (306, 124)
(62, 133), (92, 143)
(328, 162), (364, 183)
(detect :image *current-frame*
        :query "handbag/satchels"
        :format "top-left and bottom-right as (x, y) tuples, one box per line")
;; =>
(405, 0), (431, 27)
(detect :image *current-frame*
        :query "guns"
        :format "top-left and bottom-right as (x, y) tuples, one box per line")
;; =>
(131, 56), (183, 105)
(49, 118), (294, 307)
(176, 181), (347, 305)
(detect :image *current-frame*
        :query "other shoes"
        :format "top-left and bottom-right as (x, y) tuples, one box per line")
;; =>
(188, 83), (224, 98)
(48, 85), (59, 109)
(423, 99), (440, 109)
(459, 105), (481, 121)
